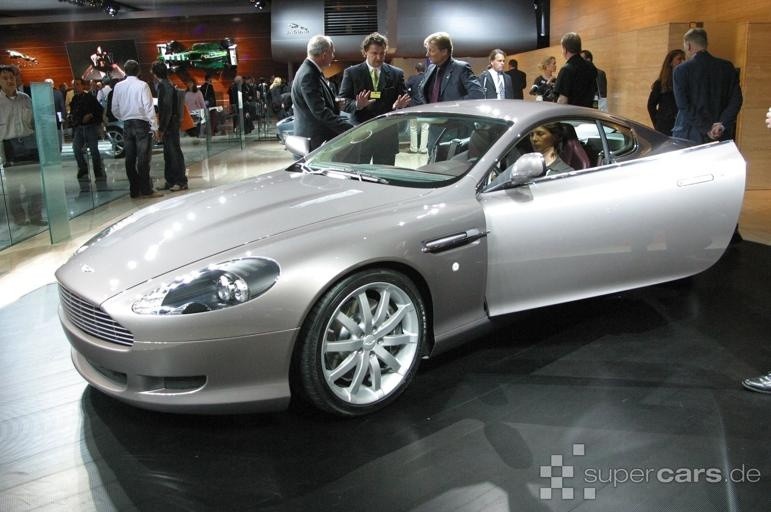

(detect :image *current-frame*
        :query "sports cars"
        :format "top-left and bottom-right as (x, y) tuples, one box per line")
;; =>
(55, 119), (164, 159)
(275, 108), (418, 159)
(52, 97), (745, 418)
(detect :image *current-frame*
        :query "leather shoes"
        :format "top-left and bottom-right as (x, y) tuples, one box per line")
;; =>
(740, 369), (770, 393)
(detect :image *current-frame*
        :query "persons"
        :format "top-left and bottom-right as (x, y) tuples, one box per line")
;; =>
(741, 99), (769, 396)
(1, 23), (744, 201)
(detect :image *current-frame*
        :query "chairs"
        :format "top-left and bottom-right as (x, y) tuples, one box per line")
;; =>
(559, 123), (590, 171)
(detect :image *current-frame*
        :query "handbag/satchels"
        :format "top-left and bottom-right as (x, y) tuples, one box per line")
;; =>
(180, 104), (194, 134)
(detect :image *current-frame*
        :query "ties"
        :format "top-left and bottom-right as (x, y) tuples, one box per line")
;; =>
(496, 72), (506, 99)
(431, 67), (443, 102)
(371, 68), (380, 91)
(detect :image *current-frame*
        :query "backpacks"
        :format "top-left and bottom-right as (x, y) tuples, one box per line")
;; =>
(166, 84), (184, 121)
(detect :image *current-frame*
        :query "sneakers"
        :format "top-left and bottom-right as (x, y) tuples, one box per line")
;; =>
(169, 184), (188, 191)
(140, 191), (163, 198)
(156, 182), (171, 190)
